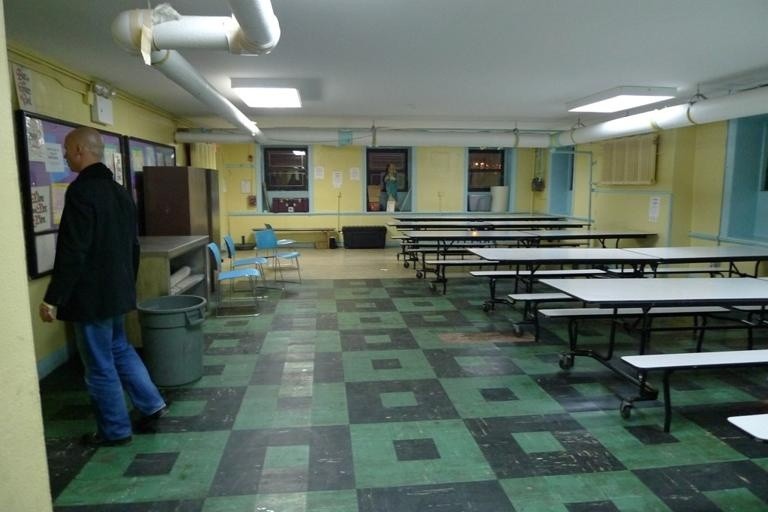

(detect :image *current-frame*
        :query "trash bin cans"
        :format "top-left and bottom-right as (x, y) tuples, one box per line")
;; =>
(136, 294), (207, 387)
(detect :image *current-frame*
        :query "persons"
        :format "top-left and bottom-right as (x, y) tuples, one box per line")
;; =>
(385, 162), (398, 212)
(40, 126), (168, 447)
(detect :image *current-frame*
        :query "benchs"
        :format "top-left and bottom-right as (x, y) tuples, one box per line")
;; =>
(385, 214), (768, 398)
(725, 414), (768, 446)
(618, 349), (768, 432)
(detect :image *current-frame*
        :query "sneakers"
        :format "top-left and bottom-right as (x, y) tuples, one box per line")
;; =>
(132, 408), (169, 426)
(83, 432), (132, 447)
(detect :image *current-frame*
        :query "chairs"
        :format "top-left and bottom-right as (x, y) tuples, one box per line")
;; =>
(208, 243), (261, 318)
(277, 238), (297, 263)
(253, 230), (302, 285)
(223, 235), (268, 299)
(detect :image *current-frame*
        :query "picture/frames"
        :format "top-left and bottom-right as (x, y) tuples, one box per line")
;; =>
(124, 134), (176, 223)
(15, 108), (127, 280)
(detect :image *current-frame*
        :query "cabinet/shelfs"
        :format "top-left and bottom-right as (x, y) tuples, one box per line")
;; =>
(206, 169), (221, 271)
(143, 165), (212, 295)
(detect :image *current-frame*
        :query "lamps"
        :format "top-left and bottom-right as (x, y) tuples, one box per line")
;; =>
(91, 80), (116, 125)
(566, 87), (678, 114)
(230, 78), (304, 109)
